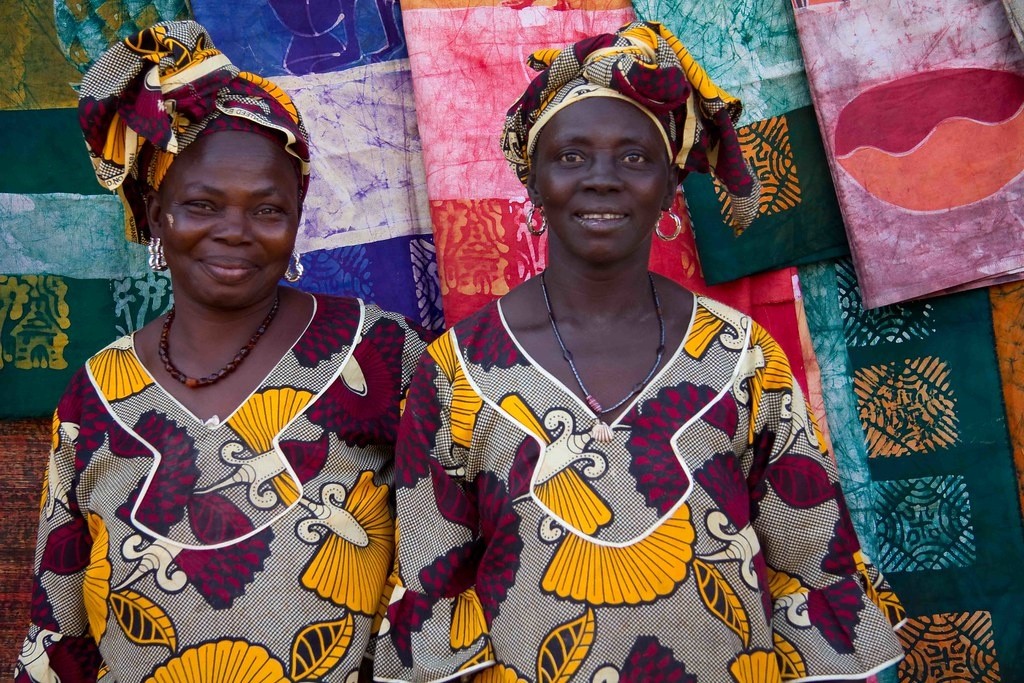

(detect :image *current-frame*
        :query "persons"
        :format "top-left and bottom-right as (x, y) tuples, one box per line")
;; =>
(14, 18), (448, 682)
(372, 21), (907, 683)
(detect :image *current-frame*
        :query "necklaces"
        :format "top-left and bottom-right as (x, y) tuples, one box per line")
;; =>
(160, 293), (280, 388)
(539, 270), (667, 415)
